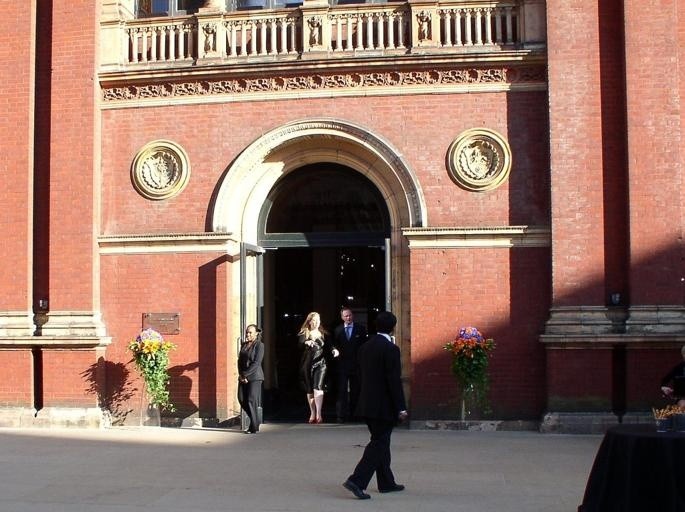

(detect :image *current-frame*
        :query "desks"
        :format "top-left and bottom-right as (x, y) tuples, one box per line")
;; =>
(577, 422), (685, 511)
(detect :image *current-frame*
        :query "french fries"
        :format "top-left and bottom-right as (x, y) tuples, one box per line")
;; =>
(651, 407), (674, 419)
(672, 404), (685, 414)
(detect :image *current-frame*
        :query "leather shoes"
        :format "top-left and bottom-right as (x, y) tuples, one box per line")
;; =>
(343, 480), (371, 499)
(379, 484), (405, 493)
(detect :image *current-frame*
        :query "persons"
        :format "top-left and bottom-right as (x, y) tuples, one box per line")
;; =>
(295, 311), (340, 424)
(236, 323), (266, 434)
(331, 306), (367, 423)
(658, 344), (685, 411)
(341, 311), (409, 499)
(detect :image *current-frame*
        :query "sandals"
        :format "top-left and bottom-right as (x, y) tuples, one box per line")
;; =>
(309, 416), (322, 424)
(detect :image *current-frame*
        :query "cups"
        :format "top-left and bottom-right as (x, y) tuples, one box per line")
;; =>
(654, 412), (685, 433)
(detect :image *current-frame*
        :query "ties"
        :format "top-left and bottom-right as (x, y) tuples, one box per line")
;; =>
(345, 327), (351, 340)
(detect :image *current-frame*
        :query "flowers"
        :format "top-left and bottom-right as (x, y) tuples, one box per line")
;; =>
(441, 327), (498, 416)
(128, 327), (176, 416)
